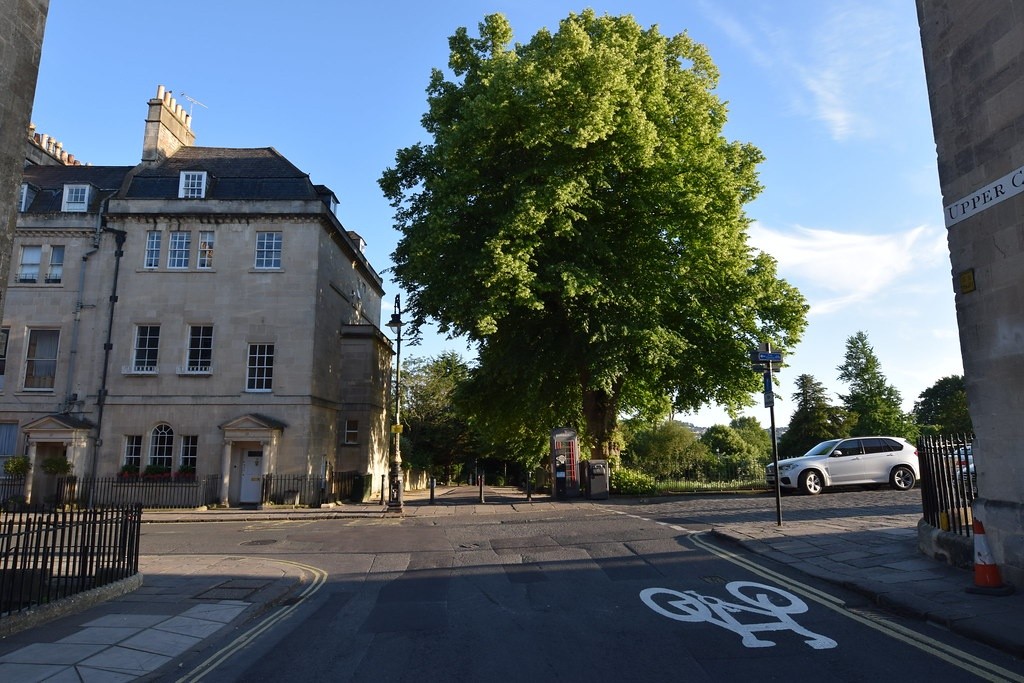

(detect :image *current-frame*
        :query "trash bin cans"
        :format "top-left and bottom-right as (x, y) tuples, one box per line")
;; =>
(429, 476), (436, 488)
(284, 490), (300, 505)
(583, 459), (609, 500)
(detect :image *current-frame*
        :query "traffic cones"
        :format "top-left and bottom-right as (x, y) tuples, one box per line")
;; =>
(969, 516), (1012, 595)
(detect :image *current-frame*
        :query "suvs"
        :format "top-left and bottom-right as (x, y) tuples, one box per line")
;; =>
(766, 435), (920, 495)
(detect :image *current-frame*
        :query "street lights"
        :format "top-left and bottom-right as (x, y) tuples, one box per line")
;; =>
(384, 292), (403, 514)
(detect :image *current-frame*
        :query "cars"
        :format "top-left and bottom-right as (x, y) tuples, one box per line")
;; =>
(945, 442), (978, 501)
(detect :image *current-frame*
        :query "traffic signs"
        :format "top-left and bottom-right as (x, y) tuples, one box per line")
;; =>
(749, 350), (781, 374)
